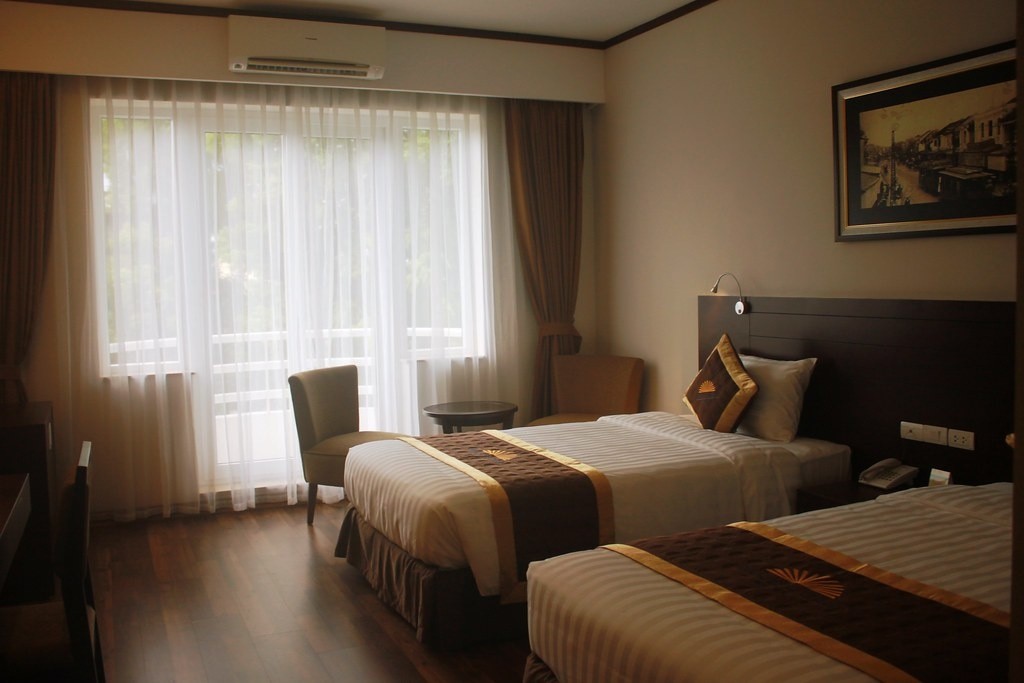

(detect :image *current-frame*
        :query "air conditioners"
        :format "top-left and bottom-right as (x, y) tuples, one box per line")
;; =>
(229, 14), (386, 81)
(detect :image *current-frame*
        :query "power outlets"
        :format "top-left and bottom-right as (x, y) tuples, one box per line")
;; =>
(948, 429), (974, 449)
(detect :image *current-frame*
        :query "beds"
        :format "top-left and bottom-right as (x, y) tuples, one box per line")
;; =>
(333, 294), (889, 655)
(528, 298), (1024, 683)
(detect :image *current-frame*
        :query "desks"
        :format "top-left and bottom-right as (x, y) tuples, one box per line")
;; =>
(422, 400), (518, 437)
(0, 473), (31, 586)
(801, 482), (908, 512)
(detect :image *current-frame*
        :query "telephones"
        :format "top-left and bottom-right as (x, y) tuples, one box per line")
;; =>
(857, 458), (919, 490)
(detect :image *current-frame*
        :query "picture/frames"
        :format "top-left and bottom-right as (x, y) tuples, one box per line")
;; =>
(830, 39), (1024, 241)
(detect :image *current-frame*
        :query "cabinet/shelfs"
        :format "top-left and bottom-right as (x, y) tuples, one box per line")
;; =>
(0, 400), (55, 530)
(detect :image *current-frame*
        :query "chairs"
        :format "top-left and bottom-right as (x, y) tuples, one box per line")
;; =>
(288, 364), (412, 524)
(0, 439), (107, 682)
(526, 352), (644, 426)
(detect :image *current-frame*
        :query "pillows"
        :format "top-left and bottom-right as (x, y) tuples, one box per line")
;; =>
(683, 334), (758, 433)
(737, 354), (817, 444)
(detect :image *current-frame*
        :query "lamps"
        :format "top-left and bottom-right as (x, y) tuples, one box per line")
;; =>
(711, 274), (746, 316)
